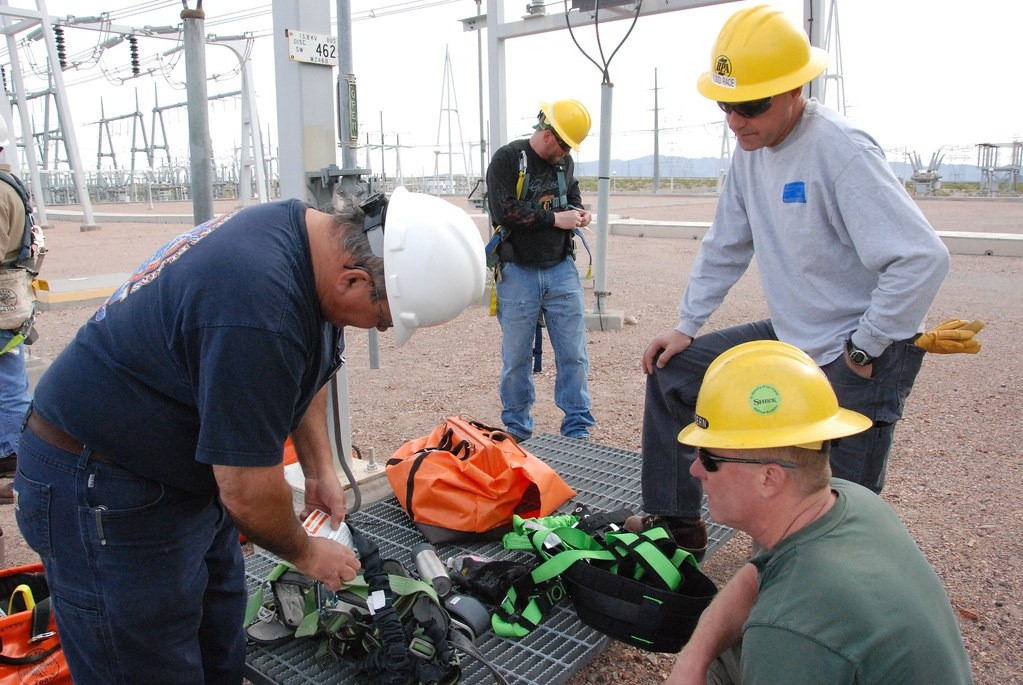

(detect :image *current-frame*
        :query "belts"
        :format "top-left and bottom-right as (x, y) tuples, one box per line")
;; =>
(26, 409), (120, 465)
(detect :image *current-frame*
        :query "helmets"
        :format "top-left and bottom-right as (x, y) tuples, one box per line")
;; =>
(697, 5), (832, 102)
(0, 114), (9, 147)
(542, 100), (591, 152)
(677, 340), (874, 449)
(383, 186), (487, 348)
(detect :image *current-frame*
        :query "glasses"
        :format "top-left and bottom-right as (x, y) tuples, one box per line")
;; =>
(717, 96), (774, 118)
(343, 265), (393, 328)
(699, 449), (798, 471)
(546, 127), (571, 151)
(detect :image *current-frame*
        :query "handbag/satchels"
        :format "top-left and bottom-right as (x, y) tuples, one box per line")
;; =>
(386, 412), (578, 544)
(0, 564), (76, 685)
(549, 534), (718, 654)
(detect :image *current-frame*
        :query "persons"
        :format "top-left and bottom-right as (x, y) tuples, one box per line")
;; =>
(486, 101), (591, 445)
(15, 186), (487, 685)
(0, 113), (36, 503)
(666, 340), (974, 685)
(624, 6), (950, 562)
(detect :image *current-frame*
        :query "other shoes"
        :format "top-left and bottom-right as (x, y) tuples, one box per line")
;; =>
(0, 480), (14, 504)
(507, 431), (524, 443)
(0, 452), (17, 478)
(623, 514), (707, 564)
(576, 437), (593, 443)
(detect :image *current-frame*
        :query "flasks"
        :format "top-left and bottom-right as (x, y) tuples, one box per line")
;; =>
(410, 542), (452, 597)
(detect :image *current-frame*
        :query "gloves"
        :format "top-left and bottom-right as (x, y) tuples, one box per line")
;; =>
(915, 317), (985, 354)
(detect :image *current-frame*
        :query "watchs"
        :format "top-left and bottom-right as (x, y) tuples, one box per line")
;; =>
(845, 329), (890, 366)
